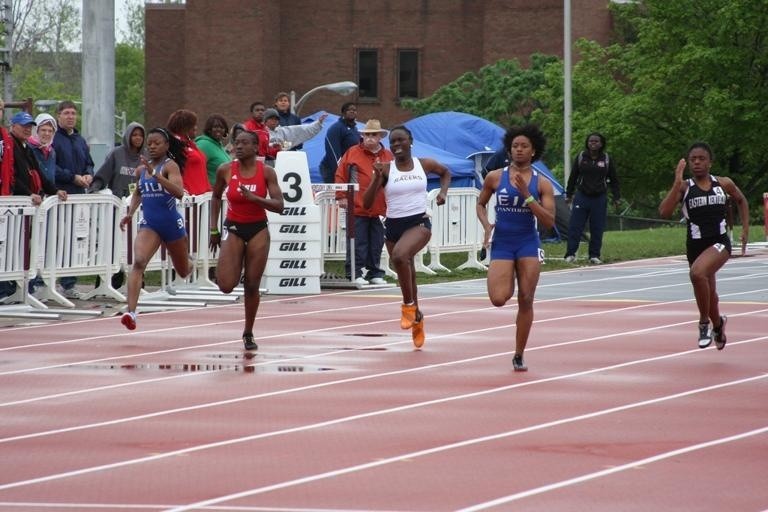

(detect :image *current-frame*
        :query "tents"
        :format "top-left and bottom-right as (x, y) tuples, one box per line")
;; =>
(390, 111), (589, 246)
(298, 111), (489, 192)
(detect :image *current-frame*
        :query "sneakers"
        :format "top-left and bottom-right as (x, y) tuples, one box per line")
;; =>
(589, 257), (602, 265)
(698, 316), (727, 350)
(243, 333), (257, 350)
(371, 278), (387, 284)
(401, 304), (425, 347)
(356, 276), (369, 284)
(565, 256), (576, 264)
(122, 312), (137, 330)
(60, 287), (81, 299)
(512, 354), (528, 370)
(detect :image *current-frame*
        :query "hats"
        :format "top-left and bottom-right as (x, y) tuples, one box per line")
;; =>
(263, 109), (280, 122)
(357, 118), (388, 138)
(12, 112), (36, 126)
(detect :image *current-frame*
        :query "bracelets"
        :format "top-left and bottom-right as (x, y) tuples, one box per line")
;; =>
(209, 230), (218, 234)
(151, 167), (155, 176)
(524, 195), (534, 204)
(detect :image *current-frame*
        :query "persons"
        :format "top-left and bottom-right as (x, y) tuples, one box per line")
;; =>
(480, 148), (509, 260)
(474, 123), (555, 370)
(86, 92), (327, 287)
(658, 142), (748, 351)
(119, 126), (194, 330)
(563, 132), (619, 265)
(361, 125), (452, 347)
(208, 131), (283, 351)
(335, 120), (394, 285)
(319, 103), (362, 247)
(0, 99), (95, 297)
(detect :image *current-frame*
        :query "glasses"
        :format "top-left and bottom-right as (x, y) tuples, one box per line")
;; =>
(365, 132), (377, 137)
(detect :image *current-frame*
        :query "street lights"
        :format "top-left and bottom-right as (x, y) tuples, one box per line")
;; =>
(287, 81), (359, 118)
(35, 98), (126, 145)
